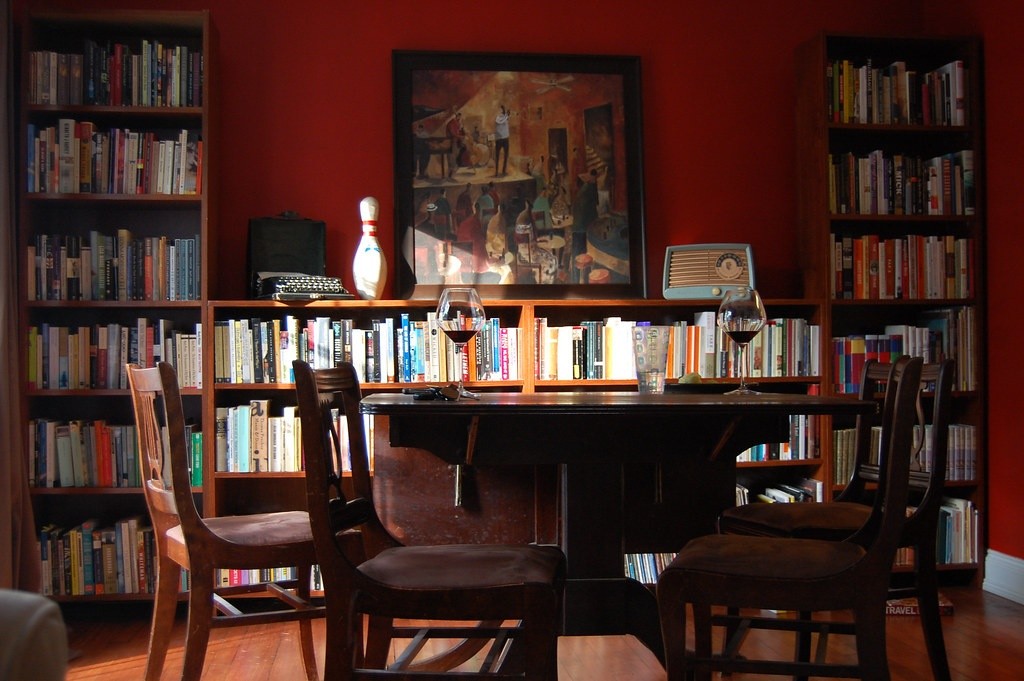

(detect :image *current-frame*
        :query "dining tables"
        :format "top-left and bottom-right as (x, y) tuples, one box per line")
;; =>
(357, 387), (868, 673)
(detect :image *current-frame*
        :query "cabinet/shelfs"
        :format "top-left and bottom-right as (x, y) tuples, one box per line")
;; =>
(206, 299), (528, 622)
(0, 7), (222, 622)
(792, 29), (988, 605)
(528, 298), (829, 622)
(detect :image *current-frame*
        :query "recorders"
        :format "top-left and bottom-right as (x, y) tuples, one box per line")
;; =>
(663, 242), (756, 299)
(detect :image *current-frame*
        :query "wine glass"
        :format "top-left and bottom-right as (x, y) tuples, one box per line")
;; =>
(717, 290), (768, 397)
(434, 288), (488, 401)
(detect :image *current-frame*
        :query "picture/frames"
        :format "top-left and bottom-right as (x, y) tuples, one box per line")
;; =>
(392, 48), (648, 299)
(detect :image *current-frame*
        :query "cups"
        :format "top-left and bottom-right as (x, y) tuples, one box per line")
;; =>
(631, 325), (671, 396)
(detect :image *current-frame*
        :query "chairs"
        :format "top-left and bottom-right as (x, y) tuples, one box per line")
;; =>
(655, 354), (923, 681)
(125, 362), (367, 679)
(716, 357), (955, 681)
(292, 359), (568, 681)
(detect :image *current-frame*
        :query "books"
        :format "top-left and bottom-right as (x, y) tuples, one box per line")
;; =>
(832, 421), (977, 485)
(892, 496), (982, 566)
(34, 512), (329, 598)
(534, 309), (820, 381)
(26, 313), (204, 390)
(214, 312), (522, 383)
(24, 116), (202, 194)
(27, 225), (202, 301)
(23, 412), (203, 490)
(826, 51), (984, 127)
(734, 382), (819, 462)
(623, 475), (826, 583)
(830, 133), (982, 215)
(215, 395), (375, 472)
(831, 304), (978, 394)
(31, 36), (203, 107)
(828, 223), (975, 300)
